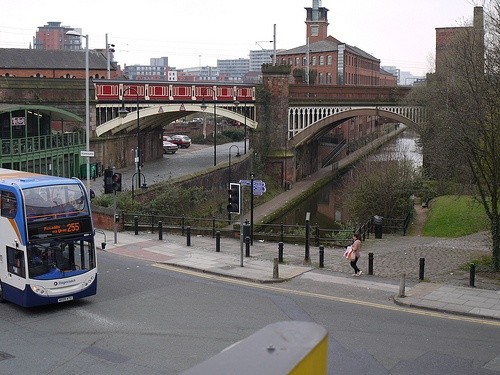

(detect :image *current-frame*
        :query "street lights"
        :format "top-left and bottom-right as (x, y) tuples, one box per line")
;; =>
(284, 131), (294, 192)
(119, 87), (142, 189)
(234, 86), (247, 156)
(250, 172), (255, 246)
(228, 145), (241, 225)
(132, 172), (149, 232)
(65, 30), (91, 201)
(201, 87), (217, 167)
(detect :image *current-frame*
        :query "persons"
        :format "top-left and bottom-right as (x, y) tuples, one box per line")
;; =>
(56, 131), (61, 146)
(52, 194), (62, 206)
(28, 254), (42, 267)
(350, 234), (362, 276)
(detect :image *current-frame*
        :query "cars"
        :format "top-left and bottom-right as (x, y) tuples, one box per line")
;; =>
(163, 141), (178, 154)
(163, 134), (192, 148)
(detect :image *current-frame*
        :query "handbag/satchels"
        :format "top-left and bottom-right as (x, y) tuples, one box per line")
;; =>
(343, 246), (356, 262)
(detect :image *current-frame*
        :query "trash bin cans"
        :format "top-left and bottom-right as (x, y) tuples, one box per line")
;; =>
(93, 162), (102, 177)
(81, 163), (97, 179)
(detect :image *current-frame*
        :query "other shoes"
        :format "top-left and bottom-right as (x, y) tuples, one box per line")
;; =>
(352, 274), (358, 277)
(357, 271), (362, 277)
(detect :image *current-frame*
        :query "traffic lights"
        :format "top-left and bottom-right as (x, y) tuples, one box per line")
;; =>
(227, 183), (240, 214)
(103, 170), (113, 194)
(113, 172), (122, 192)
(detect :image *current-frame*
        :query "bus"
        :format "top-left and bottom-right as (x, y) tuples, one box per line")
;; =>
(1, 167), (108, 310)
